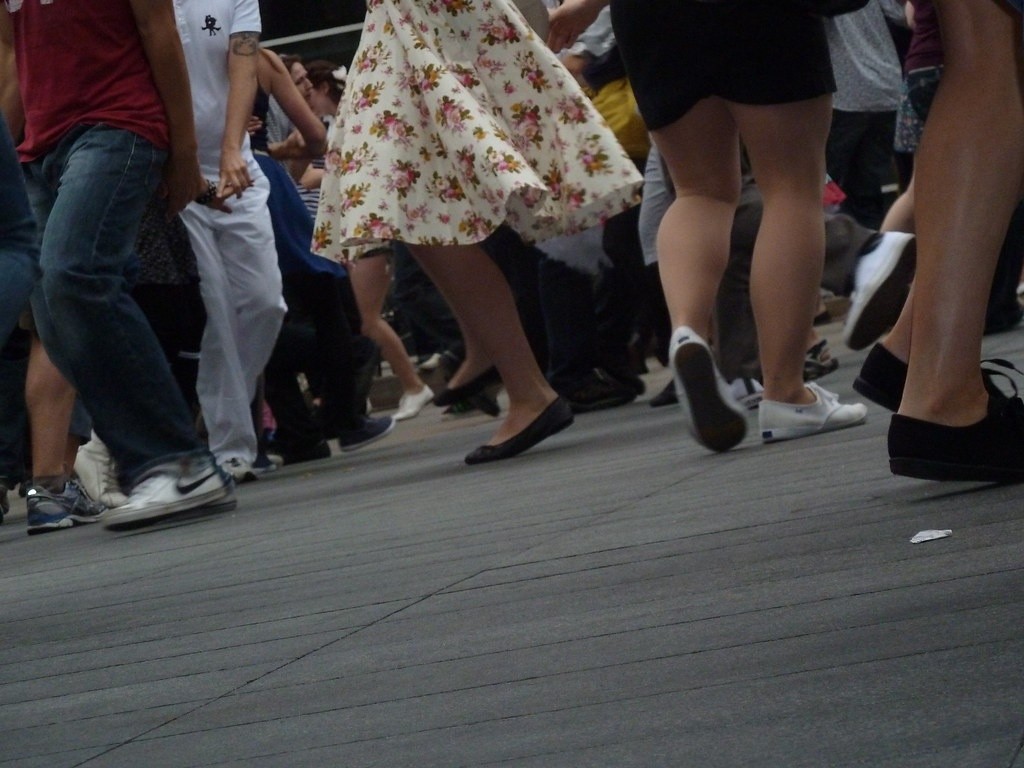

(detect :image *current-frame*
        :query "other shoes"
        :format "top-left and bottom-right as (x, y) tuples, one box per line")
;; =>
(267, 454), (284, 467)
(885, 395), (1024, 485)
(852, 341), (1024, 413)
(447, 361), (504, 403)
(262, 440), (332, 466)
(18, 479), (33, 498)
(464, 392), (576, 466)
(0, 482), (10, 515)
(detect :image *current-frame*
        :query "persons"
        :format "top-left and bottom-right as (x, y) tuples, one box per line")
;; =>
(0, 0), (1024, 535)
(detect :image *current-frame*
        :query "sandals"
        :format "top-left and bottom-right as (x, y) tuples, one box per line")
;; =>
(801, 338), (840, 381)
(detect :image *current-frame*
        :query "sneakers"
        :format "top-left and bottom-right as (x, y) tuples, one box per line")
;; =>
(250, 453), (277, 475)
(365, 397), (373, 416)
(547, 365), (637, 413)
(841, 230), (918, 351)
(667, 326), (751, 452)
(337, 414), (396, 452)
(727, 376), (765, 410)
(25, 477), (110, 536)
(757, 381), (868, 444)
(220, 456), (258, 483)
(392, 383), (437, 422)
(73, 440), (129, 508)
(101, 460), (238, 532)
